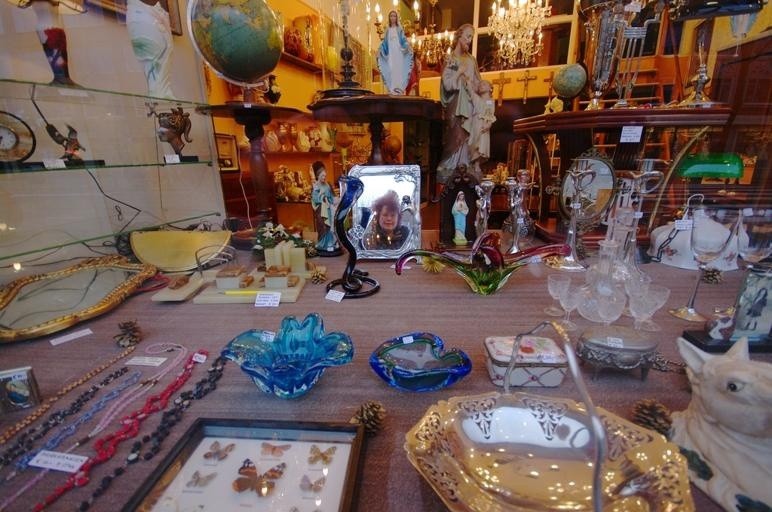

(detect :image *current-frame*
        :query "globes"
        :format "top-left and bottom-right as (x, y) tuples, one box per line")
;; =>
(185, 0), (285, 105)
(552, 62), (589, 111)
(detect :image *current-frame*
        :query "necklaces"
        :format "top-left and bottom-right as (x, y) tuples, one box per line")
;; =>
(74, 356), (226, 512)
(1, 343), (207, 512)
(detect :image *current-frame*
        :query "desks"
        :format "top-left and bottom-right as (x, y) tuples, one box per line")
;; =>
(308, 91), (436, 166)
(2, 231), (772, 510)
(512, 106), (733, 241)
(192, 101), (306, 225)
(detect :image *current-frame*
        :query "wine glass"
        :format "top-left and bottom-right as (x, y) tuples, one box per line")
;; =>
(598, 296), (623, 336)
(669, 212), (772, 322)
(546, 272), (580, 331)
(626, 273), (669, 333)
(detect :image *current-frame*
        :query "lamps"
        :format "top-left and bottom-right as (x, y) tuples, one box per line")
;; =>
(488, 0), (554, 69)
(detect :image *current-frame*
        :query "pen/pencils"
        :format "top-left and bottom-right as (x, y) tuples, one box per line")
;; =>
(218, 290), (280, 295)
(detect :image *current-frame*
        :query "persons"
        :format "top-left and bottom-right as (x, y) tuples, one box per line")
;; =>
(377, 11), (413, 95)
(363, 190), (411, 250)
(440, 23), (496, 172)
(743, 288), (768, 331)
(452, 191), (469, 241)
(310, 161), (338, 252)
(474, 181), (492, 243)
(508, 186), (527, 255)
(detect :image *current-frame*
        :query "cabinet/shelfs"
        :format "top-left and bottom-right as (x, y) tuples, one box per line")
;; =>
(0, 76), (222, 261)
(245, 2), (368, 205)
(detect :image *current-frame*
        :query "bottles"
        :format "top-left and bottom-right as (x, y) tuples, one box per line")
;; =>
(576, 239), (626, 322)
